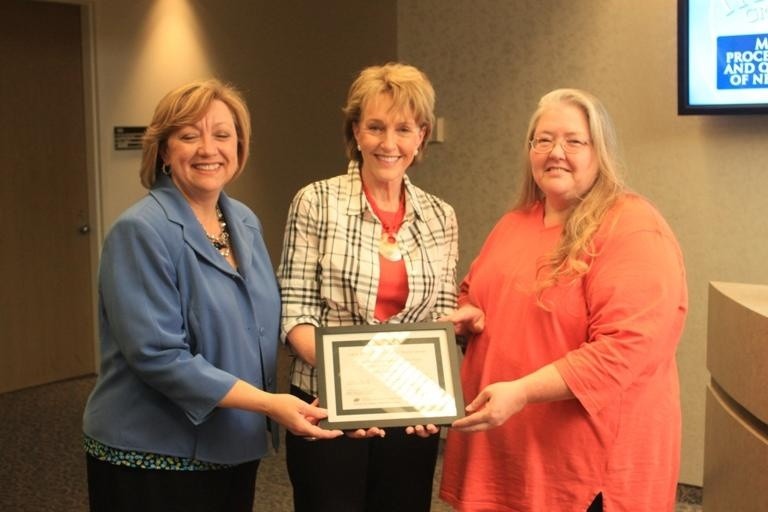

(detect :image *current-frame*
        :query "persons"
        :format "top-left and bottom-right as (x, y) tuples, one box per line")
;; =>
(438, 88), (688, 512)
(82, 79), (344, 512)
(277, 60), (458, 512)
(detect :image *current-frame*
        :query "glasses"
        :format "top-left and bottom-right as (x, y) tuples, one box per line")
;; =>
(528, 135), (597, 156)
(355, 120), (421, 137)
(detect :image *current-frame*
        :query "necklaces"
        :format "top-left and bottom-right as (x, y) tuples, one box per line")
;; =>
(193, 205), (230, 251)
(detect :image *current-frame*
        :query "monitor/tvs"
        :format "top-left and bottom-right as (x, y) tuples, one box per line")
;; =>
(677, 0), (768, 116)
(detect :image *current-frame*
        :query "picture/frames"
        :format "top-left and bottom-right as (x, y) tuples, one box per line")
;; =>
(315, 321), (465, 430)
